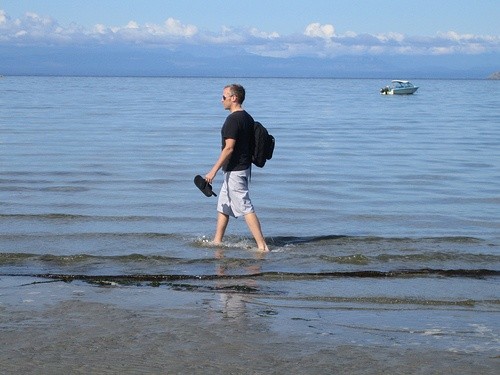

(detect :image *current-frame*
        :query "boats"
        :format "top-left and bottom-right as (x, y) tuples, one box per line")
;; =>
(379, 80), (419, 95)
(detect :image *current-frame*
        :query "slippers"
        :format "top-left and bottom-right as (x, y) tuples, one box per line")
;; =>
(194, 174), (212, 197)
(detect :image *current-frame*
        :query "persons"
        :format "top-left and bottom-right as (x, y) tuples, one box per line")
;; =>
(204, 84), (270, 252)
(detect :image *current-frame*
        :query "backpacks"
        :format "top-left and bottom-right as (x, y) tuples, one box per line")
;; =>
(251, 122), (275, 168)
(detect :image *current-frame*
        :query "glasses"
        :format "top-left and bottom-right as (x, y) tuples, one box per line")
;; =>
(222, 95), (234, 100)
